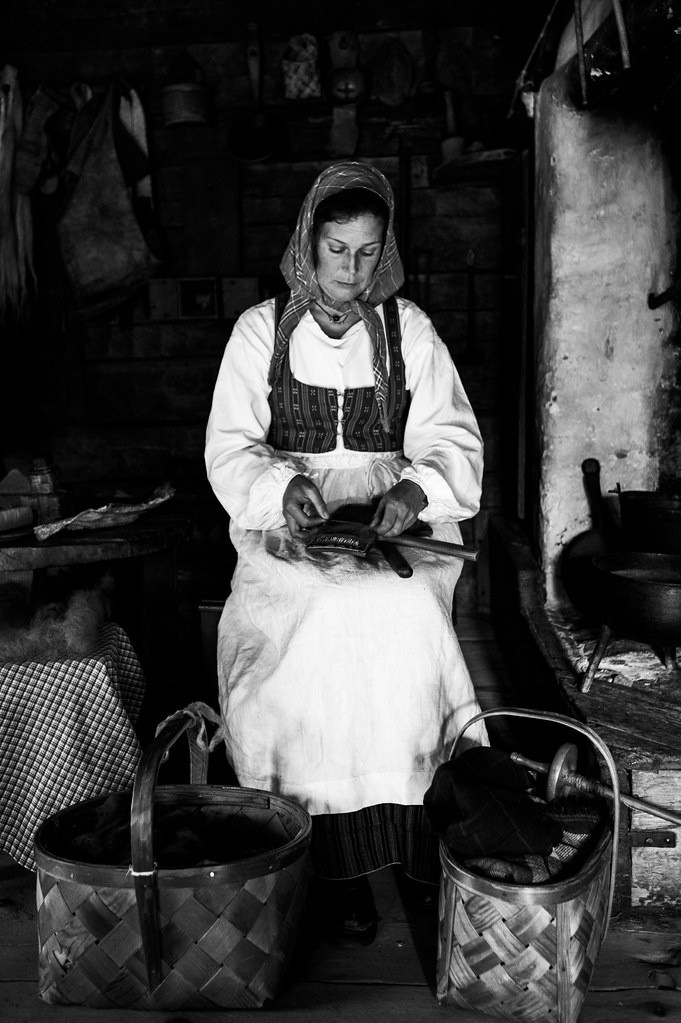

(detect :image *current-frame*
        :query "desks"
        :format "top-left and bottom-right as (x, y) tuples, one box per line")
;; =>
(0, 623), (146, 873)
(0, 513), (198, 617)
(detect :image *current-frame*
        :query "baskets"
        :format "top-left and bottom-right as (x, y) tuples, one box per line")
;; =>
(436, 707), (630, 1023)
(34, 702), (312, 1013)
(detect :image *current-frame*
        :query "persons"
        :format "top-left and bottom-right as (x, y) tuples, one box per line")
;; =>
(204, 162), (491, 937)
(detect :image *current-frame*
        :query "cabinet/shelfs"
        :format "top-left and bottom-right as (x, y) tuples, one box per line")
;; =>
(0, 0), (538, 638)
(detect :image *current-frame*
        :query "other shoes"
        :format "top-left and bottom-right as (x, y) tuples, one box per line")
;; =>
(326, 862), (379, 944)
(409, 875), (436, 953)
